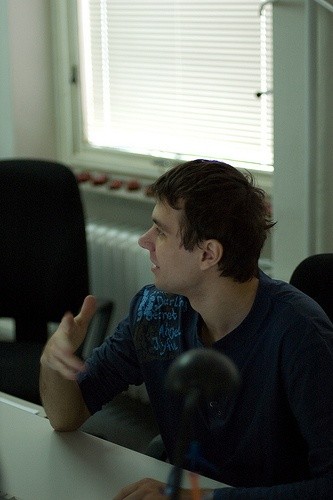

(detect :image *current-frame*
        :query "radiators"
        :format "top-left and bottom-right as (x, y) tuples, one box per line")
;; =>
(86, 225), (270, 403)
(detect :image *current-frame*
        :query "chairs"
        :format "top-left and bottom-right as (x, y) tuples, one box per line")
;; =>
(0, 160), (113, 405)
(141, 253), (333, 478)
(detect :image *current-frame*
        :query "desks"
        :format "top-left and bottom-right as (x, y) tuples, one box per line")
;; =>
(0, 391), (235, 500)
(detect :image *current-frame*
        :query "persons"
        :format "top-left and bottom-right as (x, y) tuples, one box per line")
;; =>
(39, 158), (333, 500)
